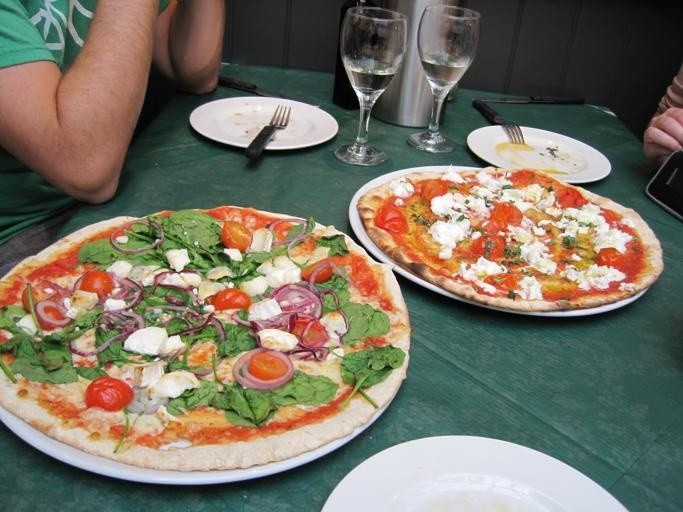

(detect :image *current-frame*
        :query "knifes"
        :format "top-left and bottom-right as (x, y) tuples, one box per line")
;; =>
(479, 95), (587, 104)
(217, 75), (320, 109)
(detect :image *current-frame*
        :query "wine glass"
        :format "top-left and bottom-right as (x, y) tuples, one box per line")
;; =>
(332, 5), (408, 168)
(417, 5), (482, 155)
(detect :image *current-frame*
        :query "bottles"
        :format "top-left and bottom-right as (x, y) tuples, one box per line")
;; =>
(331, 1), (390, 109)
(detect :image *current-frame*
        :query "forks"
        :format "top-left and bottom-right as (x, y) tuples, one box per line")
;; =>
(245, 104), (291, 160)
(472, 99), (525, 145)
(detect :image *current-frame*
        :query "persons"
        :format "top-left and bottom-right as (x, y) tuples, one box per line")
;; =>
(641, 62), (683, 172)
(0, 0), (228, 280)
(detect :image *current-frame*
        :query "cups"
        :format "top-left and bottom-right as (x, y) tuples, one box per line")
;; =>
(371, 1), (467, 129)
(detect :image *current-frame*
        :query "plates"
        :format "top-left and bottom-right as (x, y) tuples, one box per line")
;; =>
(316, 433), (626, 512)
(188, 94), (338, 152)
(466, 124), (611, 184)
